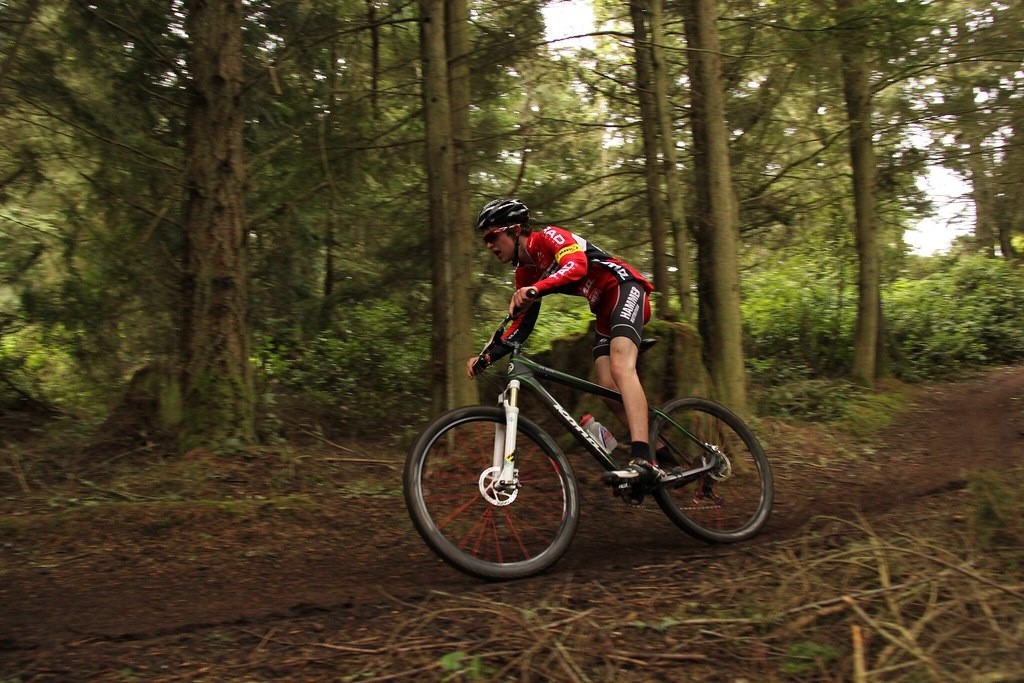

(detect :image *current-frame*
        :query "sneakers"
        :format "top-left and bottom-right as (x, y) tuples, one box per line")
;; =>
(662, 465), (691, 485)
(598, 458), (660, 486)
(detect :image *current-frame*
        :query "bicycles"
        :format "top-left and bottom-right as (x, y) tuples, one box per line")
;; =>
(402, 289), (776, 585)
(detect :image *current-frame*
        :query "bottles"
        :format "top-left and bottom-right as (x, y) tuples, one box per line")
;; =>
(580, 414), (617, 452)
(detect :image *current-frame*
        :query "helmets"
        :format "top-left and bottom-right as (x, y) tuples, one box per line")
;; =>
(476, 199), (530, 230)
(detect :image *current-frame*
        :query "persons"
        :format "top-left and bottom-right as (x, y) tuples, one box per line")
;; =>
(466, 199), (668, 485)
